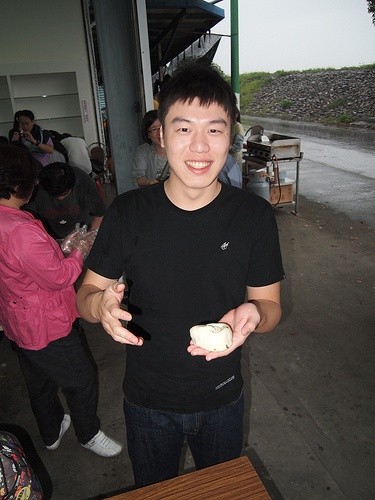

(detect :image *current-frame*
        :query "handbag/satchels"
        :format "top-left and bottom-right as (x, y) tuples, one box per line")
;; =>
(0, 431), (44, 500)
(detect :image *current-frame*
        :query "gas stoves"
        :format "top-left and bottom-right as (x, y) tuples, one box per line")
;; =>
(247, 132), (301, 161)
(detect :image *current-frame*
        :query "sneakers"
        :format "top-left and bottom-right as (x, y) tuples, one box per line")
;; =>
(80, 429), (122, 457)
(46, 415), (70, 449)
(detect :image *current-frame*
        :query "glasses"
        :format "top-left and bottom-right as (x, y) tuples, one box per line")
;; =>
(148, 128), (160, 133)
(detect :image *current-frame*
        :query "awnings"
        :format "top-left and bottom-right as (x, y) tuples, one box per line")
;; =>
(89, 0), (224, 88)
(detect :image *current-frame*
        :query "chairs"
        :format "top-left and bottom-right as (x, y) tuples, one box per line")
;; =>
(86, 142), (109, 198)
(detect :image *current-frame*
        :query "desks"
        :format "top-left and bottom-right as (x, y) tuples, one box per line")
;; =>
(103, 455), (272, 500)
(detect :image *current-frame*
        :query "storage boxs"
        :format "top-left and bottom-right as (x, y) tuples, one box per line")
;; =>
(269, 183), (293, 205)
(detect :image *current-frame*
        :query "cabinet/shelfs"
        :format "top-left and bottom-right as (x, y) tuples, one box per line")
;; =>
(0, 59), (99, 156)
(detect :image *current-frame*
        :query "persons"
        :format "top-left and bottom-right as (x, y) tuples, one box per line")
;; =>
(0, 110), (104, 258)
(0, 138), (123, 459)
(132, 77), (244, 189)
(76, 64), (286, 489)
